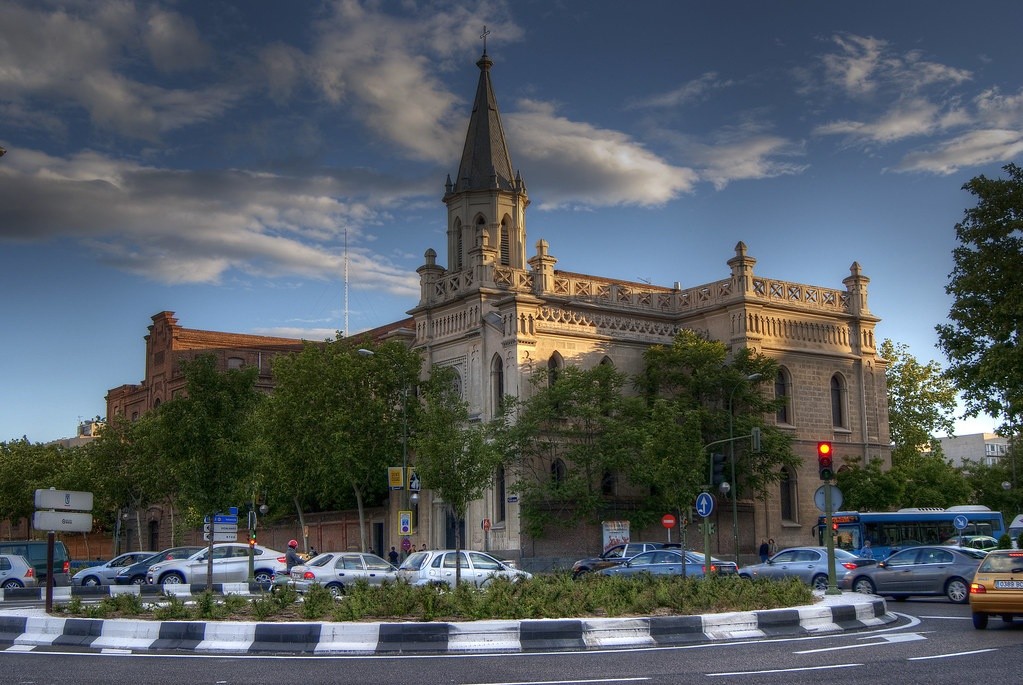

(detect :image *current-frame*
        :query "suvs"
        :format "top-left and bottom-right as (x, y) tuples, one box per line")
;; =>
(571, 540), (682, 581)
(0, 541), (71, 588)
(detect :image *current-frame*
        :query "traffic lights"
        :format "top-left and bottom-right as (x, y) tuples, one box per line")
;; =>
(713, 454), (726, 484)
(247, 530), (256, 544)
(817, 442), (834, 481)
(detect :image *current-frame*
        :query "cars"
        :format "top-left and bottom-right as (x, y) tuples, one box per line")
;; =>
(969, 548), (1023, 629)
(940, 535), (999, 553)
(72, 551), (160, 593)
(145, 542), (287, 592)
(114, 546), (207, 589)
(288, 549), (532, 601)
(0, 555), (37, 596)
(591, 546), (989, 604)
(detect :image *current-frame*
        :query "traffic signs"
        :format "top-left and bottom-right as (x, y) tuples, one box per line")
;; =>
(204, 507), (237, 541)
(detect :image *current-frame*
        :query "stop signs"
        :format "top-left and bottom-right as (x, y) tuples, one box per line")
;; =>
(662, 515), (676, 528)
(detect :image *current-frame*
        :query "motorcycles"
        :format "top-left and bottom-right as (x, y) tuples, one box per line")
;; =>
(269, 552), (313, 597)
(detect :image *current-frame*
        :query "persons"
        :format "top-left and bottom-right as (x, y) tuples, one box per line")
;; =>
(407, 545), (416, 557)
(368, 547), (375, 554)
(859, 541), (874, 559)
(388, 547), (398, 567)
(286, 540), (304, 575)
(759, 539), (768, 563)
(309, 547), (314, 556)
(418, 544), (426, 552)
(768, 539), (777, 557)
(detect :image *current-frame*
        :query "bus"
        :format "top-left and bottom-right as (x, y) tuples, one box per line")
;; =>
(812, 504), (1004, 551)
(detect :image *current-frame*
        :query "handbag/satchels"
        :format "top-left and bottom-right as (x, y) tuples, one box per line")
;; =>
(768, 551), (773, 556)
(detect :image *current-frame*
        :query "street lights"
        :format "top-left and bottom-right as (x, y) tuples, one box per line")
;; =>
(730, 373), (762, 564)
(358, 349), (408, 558)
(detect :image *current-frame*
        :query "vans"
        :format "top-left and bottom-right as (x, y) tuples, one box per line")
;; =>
(1007, 514), (1023, 549)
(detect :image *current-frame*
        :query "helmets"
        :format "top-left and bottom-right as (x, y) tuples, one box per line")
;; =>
(289, 540), (298, 549)
